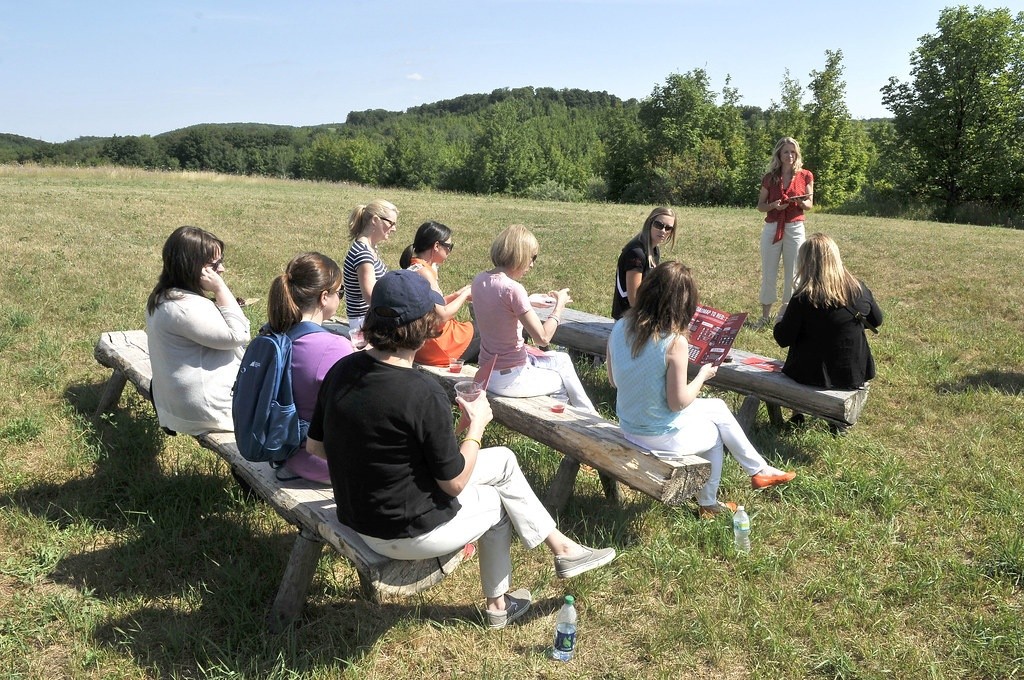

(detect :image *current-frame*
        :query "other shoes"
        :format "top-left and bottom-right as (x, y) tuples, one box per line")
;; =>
(555, 545), (615, 578)
(752, 471), (796, 488)
(700, 502), (736, 515)
(822, 424), (846, 436)
(791, 414), (805, 431)
(486, 588), (531, 630)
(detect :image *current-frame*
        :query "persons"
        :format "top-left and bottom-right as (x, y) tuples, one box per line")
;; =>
(306, 269), (616, 631)
(400, 221), (481, 368)
(754, 137), (813, 328)
(612, 207), (678, 321)
(146, 226), (251, 436)
(471, 224), (597, 414)
(606, 261), (796, 518)
(773, 232), (883, 438)
(267, 253), (373, 485)
(342, 199), (399, 330)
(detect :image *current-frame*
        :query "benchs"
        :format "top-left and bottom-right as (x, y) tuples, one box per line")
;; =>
(469, 300), (870, 442)
(323, 317), (714, 520)
(92, 329), (466, 635)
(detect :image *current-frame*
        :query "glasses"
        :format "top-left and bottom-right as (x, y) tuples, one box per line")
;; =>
(335, 285), (345, 299)
(653, 220), (674, 231)
(380, 217), (395, 227)
(439, 242), (453, 251)
(207, 254), (224, 271)
(533, 255), (537, 262)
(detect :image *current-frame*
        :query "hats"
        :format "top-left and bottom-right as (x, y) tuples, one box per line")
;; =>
(371, 270), (445, 325)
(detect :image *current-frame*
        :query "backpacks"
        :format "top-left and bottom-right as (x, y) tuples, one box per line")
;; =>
(232, 321), (328, 462)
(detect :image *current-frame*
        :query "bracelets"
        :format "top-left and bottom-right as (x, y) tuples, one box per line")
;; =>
(548, 314), (561, 326)
(458, 438), (481, 448)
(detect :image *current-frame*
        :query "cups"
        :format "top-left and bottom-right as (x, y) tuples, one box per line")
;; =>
(349, 328), (368, 350)
(723, 345), (733, 362)
(449, 358), (465, 373)
(550, 395), (570, 413)
(454, 381), (482, 409)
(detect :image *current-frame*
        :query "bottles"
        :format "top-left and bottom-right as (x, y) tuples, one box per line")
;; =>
(553, 595), (577, 661)
(734, 506), (750, 553)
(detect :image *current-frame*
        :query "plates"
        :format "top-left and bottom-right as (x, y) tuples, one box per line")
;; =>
(531, 297), (557, 306)
(789, 194), (812, 200)
